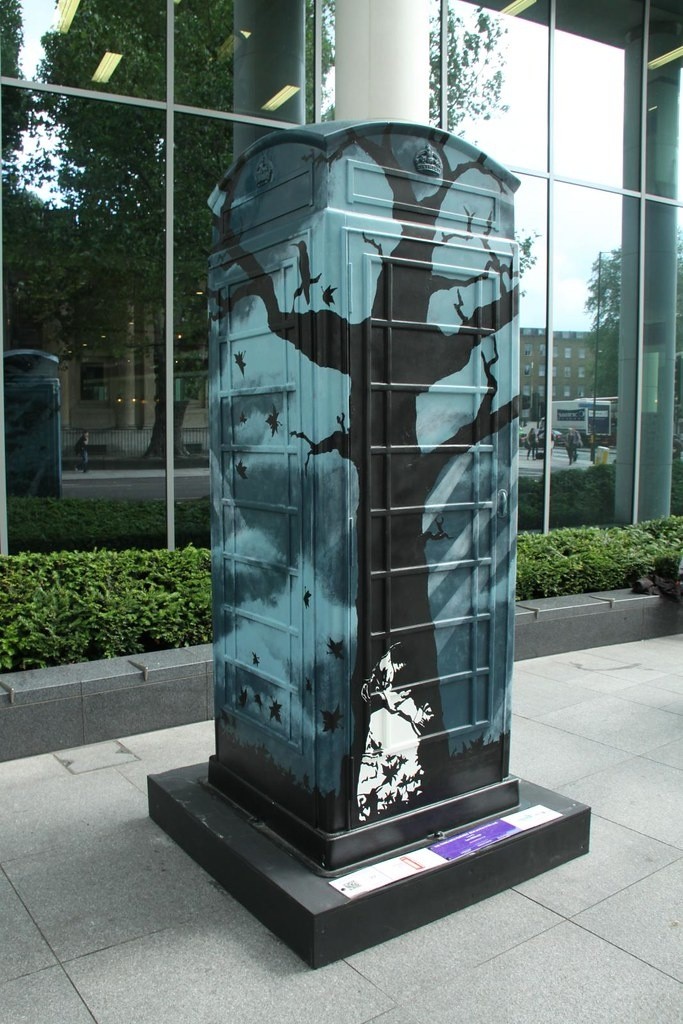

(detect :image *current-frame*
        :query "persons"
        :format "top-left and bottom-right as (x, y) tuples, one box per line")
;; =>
(526, 426), (541, 461)
(74, 429), (90, 474)
(552, 428), (583, 466)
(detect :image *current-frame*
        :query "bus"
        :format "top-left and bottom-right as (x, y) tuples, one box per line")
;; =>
(575, 396), (617, 446)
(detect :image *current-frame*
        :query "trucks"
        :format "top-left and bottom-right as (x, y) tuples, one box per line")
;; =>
(537, 399), (612, 447)
